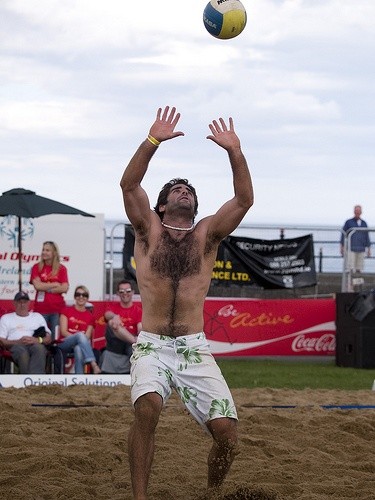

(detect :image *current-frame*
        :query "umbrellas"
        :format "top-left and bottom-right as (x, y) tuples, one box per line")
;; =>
(0, 188), (96, 292)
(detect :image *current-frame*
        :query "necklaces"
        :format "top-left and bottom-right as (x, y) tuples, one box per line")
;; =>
(162, 223), (194, 231)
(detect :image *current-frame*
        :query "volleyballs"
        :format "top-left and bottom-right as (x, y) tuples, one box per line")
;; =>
(203, 0), (247, 40)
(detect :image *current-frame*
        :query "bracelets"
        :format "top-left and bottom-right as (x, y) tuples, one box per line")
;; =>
(39, 337), (42, 343)
(148, 134), (160, 146)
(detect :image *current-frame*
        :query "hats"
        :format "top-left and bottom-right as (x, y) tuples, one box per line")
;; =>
(14, 292), (30, 301)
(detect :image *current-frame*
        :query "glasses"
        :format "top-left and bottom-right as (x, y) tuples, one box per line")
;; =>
(75, 292), (88, 298)
(119, 289), (132, 295)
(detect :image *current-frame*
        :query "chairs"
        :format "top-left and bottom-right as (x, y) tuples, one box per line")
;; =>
(0, 311), (131, 374)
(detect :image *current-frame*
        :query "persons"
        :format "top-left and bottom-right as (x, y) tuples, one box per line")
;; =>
(29, 241), (68, 338)
(339, 206), (371, 273)
(58, 285), (102, 374)
(0, 292), (52, 375)
(103, 281), (142, 356)
(119, 106), (254, 500)
